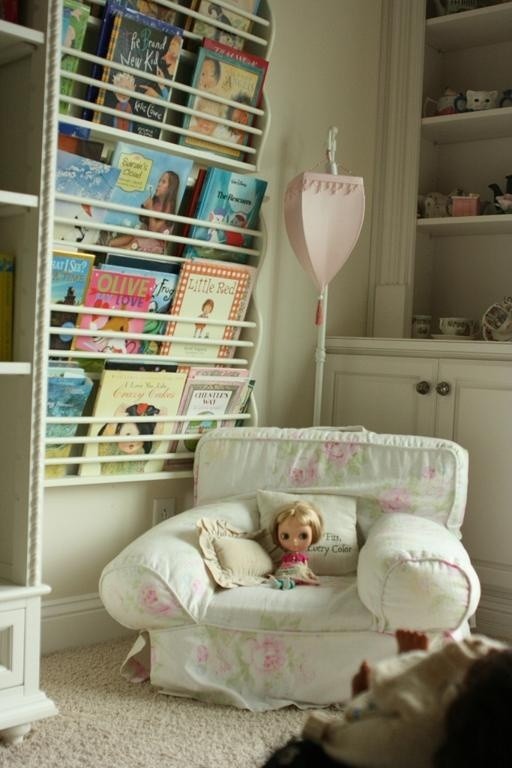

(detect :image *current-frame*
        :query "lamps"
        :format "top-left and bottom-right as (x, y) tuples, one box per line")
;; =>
(282, 126), (366, 429)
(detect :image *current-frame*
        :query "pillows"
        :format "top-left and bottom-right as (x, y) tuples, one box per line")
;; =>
(254, 488), (359, 577)
(194, 515), (290, 591)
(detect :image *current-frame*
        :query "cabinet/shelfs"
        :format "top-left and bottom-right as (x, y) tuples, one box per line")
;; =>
(0, 3), (59, 741)
(318, 352), (511, 598)
(391, 0), (511, 344)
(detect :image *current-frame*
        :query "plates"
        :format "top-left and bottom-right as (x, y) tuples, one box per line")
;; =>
(429, 331), (479, 340)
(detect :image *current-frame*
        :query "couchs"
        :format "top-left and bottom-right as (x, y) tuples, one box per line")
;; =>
(94, 425), (484, 712)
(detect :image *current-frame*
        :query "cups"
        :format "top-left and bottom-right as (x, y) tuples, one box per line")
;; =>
(411, 313), (431, 337)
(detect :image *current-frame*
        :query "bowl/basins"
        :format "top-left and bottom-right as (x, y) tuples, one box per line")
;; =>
(439, 316), (471, 335)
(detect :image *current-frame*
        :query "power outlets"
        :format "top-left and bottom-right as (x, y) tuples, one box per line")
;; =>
(149, 497), (177, 530)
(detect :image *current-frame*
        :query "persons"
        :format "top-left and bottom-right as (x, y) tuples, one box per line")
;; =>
(268, 498), (325, 589)
